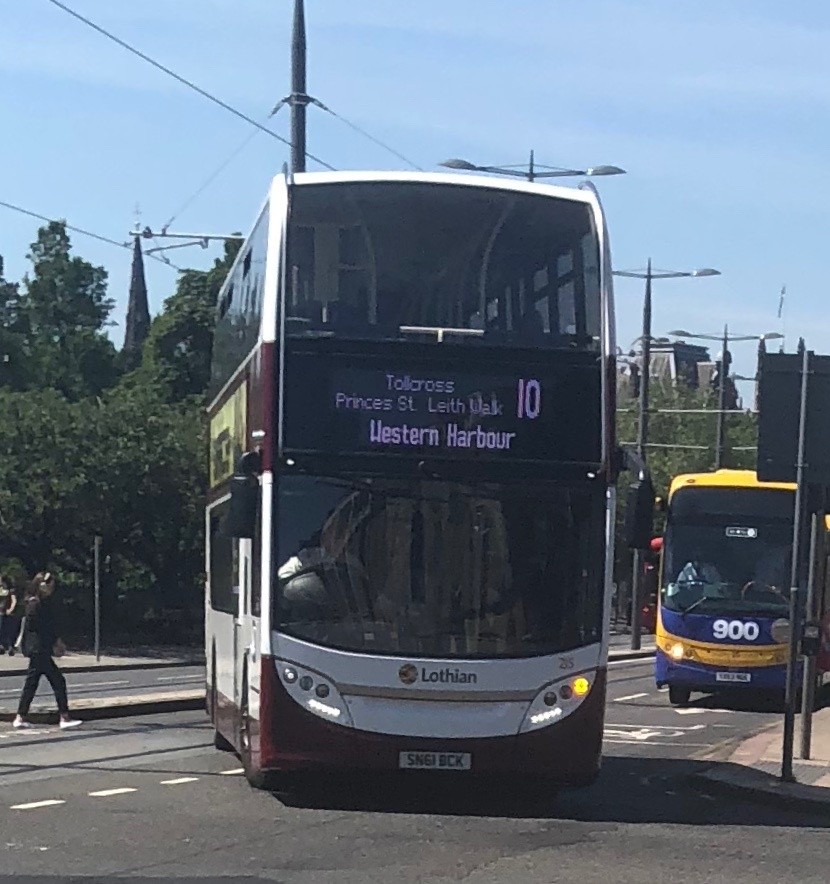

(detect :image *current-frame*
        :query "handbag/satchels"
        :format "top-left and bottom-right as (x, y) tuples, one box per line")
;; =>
(51, 641), (66, 656)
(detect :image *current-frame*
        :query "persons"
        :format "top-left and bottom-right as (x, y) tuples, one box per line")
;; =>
(0, 589), (17, 655)
(279, 511), (429, 627)
(12, 572), (84, 729)
(677, 548), (721, 584)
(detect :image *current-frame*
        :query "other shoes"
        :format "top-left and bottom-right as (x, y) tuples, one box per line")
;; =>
(60, 720), (83, 730)
(12, 719), (31, 728)
(0, 647), (5, 655)
(9, 649), (15, 656)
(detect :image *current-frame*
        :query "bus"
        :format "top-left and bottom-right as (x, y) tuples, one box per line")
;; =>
(653, 468), (829, 708)
(202, 161), (657, 811)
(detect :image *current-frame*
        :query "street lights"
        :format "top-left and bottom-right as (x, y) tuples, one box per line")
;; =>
(438, 149), (625, 178)
(667, 327), (785, 471)
(610, 268), (722, 648)
